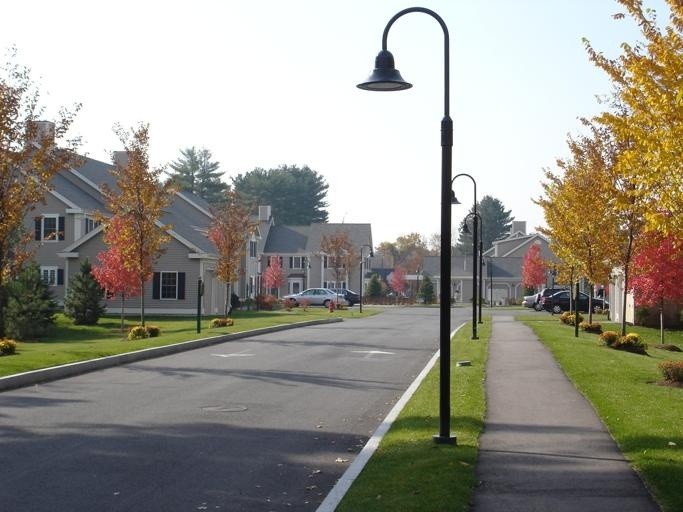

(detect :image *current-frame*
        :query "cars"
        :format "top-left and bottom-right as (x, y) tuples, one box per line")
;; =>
(330, 288), (361, 307)
(522, 285), (609, 313)
(280, 288), (347, 308)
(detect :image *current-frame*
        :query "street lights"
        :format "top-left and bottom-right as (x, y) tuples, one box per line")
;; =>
(451, 173), (493, 339)
(359, 242), (375, 313)
(416, 263), (420, 299)
(356, 6), (457, 448)
